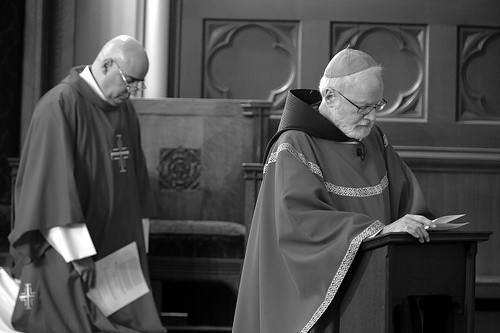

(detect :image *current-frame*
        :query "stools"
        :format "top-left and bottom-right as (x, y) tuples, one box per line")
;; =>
(148, 217), (246, 331)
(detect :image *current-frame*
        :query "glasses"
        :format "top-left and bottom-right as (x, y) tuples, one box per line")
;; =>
(333, 89), (388, 115)
(111, 61), (147, 93)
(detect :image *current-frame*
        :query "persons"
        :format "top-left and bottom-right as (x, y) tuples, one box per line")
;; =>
(8, 34), (166, 333)
(232, 44), (437, 333)
